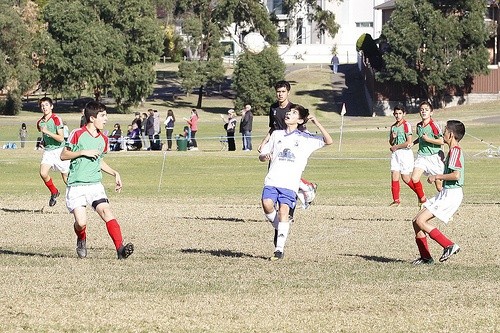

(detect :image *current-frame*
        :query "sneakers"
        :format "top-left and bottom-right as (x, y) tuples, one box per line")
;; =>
(117, 242), (134, 260)
(274, 228), (278, 247)
(300, 203), (309, 210)
(411, 258), (433, 265)
(49, 192), (60, 207)
(304, 183), (317, 204)
(76, 237), (87, 258)
(439, 244), (461, 262)
(268, 250), (284, 261)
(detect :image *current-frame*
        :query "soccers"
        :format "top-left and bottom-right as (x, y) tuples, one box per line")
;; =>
(243, 33), (265, 55)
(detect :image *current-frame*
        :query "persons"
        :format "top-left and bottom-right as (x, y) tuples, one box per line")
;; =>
(331, 54), (339, 73)
(407, 100), (445, 208)
(411, 121), (465, 263)
(239, 105), (254, 150)
(126, 109), (163, 151)
(175, 108), (200, 151)
(388, 105), (427, 208)
(19, 123), (27, 148)
(37, 109), (87, 149)
(258, 104), (334, 262)
(220, 109), (237, 151)
(108, 123), (121, 151)
(164, 110), (175, 151)
(258, 80), (318, 220)
(60, 102), (133, 260)
(37, 97), (68, 206)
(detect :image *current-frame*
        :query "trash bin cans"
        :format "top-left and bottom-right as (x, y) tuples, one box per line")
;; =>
(177, 139), (188, 151)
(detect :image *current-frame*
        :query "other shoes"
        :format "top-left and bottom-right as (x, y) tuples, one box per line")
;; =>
(168, 148), (171, 151)
(389, 201), (400, 207)
(191, 147), (198, 151)
(419, 198), (428, 210)
